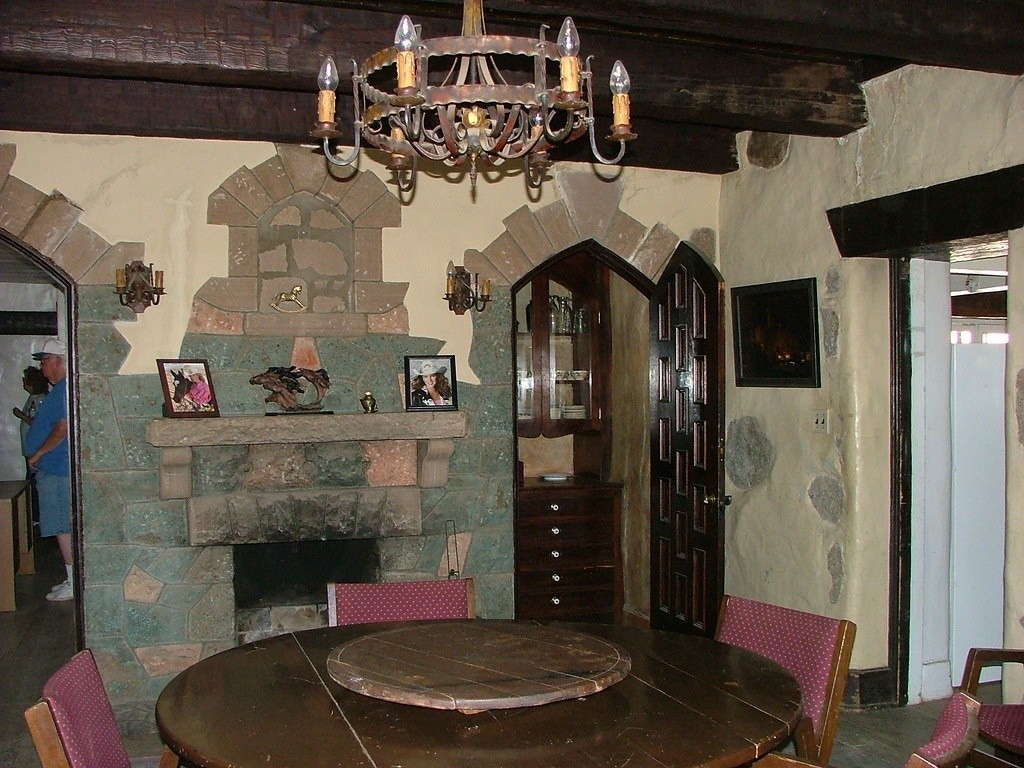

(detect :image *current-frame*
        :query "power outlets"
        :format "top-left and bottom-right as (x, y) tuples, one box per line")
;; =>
(812, 409), (830, 434)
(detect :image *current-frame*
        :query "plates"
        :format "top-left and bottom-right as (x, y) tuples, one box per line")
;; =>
(561, 405), (587, 420)
(540, 473), (572, 481)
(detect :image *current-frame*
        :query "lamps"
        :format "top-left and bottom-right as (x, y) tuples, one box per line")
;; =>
(308, 0), (638, 192)
(112, 261), (167, 313)
(442, 260), (493, 315)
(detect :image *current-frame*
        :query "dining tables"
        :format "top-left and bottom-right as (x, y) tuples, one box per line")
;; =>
(155, 619), (821, 768)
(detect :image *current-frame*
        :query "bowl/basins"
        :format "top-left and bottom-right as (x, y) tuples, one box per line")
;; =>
(556, 370), (566, 380)
(571, 371), (588, 381)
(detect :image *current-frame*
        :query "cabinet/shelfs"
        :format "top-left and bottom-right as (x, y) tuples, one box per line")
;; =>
(513, 263), (601, 438)
(513, 475), (625, 624)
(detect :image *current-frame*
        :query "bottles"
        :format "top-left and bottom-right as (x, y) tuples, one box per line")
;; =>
(574, 308), (588, 333)
(557, 297), (572, 334)
(549, 295), (559, 334)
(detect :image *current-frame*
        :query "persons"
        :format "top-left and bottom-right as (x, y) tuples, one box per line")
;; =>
(410, 359), (452, 406)
(24, 338), (73, 602)
(11, 366), (48, 524)
(187, 370), (214, 412)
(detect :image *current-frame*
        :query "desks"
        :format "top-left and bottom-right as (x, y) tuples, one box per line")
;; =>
(0, 480), (36, 613)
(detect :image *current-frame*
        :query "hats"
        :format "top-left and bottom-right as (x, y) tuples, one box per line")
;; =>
(31, 339), (67, 355)
(186, 367), (200, 376)
(412, 359), (447, 377)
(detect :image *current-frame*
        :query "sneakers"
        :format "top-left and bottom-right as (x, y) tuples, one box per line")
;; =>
(46, 579), (74, 602)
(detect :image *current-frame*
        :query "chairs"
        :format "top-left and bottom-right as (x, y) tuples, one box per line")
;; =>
(326, 578), (476, 628)
(25, 648), (132, 768)
(713, 594), (857, 768)
(754, 691), (982, 768)
(959, 648), (1024, 768)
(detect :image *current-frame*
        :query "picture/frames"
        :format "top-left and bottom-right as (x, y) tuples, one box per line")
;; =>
(730, 276), (822, 388)
(404, 354), (458, 412)
(156, 359), (221, 418)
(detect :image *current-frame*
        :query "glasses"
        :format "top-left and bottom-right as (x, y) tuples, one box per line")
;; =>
(40, 357), (50, 365)
